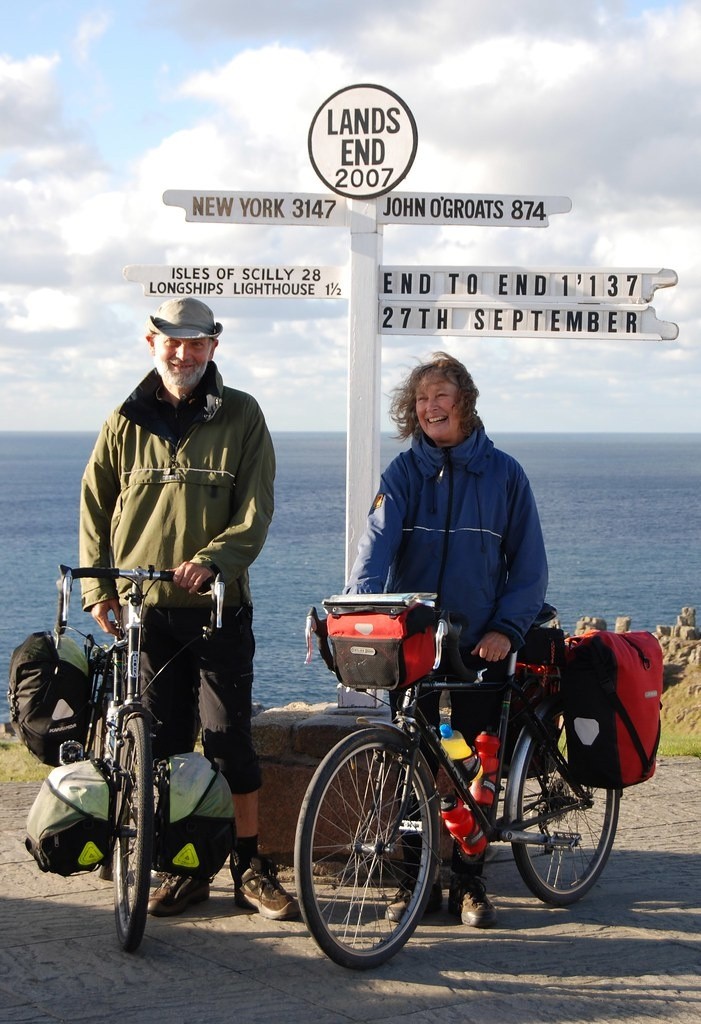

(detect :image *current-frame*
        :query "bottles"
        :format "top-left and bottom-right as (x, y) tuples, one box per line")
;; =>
(440, 792), (488, 855)
(438, 724), (483, 783)
(471, 727), (501, 805)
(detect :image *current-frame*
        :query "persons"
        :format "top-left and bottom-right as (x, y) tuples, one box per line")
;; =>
(343, 352), (549, 928)
(79, 297), (299, 920)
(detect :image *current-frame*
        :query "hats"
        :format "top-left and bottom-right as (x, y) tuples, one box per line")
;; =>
(147, 297), (223, 340)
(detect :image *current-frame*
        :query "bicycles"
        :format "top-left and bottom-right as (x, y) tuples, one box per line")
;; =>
(293, 601), (624, 972)
(55, 562), (217, 955)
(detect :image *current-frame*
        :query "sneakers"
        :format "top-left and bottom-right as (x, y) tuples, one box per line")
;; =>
(448, 870), (498, 927)
(147, 874), (210, 916)
(230, 857), (301, 920)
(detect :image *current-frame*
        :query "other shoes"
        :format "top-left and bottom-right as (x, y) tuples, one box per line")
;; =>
(385, 877), (443, 922)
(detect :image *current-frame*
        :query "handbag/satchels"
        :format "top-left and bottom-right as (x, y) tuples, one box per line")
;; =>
(488, 626), (559, 778)
(25, 760), (115, 877)
(325, 597), (436, 688)
(153, 752), (236, 882)
(563, 632), (664, 789)
(7, 631), (93, 767)
